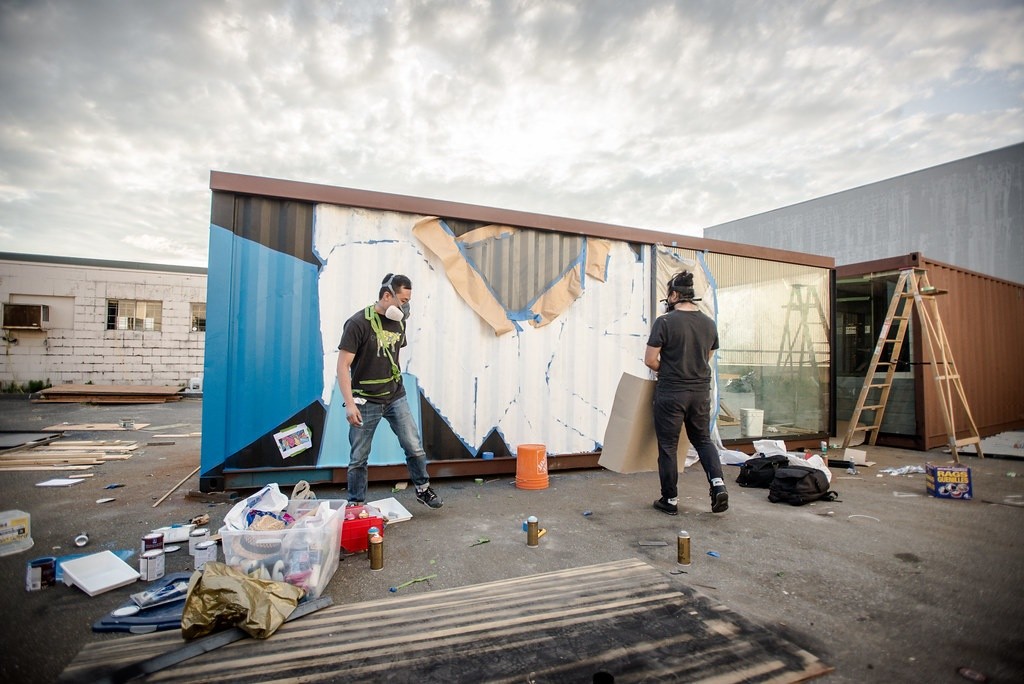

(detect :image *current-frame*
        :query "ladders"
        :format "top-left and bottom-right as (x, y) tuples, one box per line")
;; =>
(842, 267), (985, 466)
(773, 284), (833, 430)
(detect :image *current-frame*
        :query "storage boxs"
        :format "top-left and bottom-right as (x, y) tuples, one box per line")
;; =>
(219, 498), (348, 601)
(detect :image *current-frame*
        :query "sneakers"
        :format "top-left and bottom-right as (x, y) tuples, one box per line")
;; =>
(415, 488), (443, 511)
(708, 478), (730, 514)
(653, 496), (678, 515)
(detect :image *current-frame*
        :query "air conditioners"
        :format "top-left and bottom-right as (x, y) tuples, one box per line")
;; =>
(0, 302), (50, 330)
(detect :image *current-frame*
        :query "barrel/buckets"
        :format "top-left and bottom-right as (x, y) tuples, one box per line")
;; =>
(515, 444), (550, 490)
(25, 556), (54, 592)
(194, 539), (217, 571)
(139, 549), (165, 581)
(740, 407), (763, 436)
(141, 532), (164, 554)
(189, 528), (210, 556)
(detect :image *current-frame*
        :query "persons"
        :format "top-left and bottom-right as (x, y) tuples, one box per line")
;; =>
(644, 269), (732, 516)
(336, 273), (444, 512)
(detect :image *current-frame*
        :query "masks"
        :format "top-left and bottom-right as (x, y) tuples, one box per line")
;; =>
(398, 302), (411, 322)
(657, 299), (674, 313)
